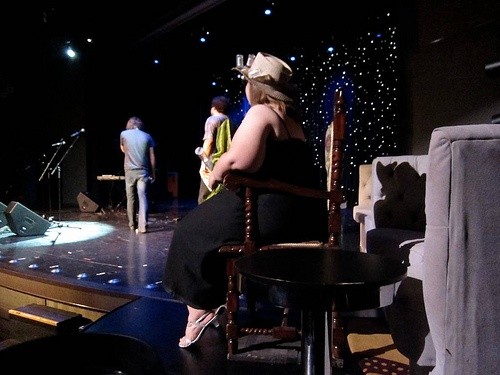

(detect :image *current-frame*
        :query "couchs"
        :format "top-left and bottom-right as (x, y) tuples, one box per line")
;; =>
(353, 123), (500, 375)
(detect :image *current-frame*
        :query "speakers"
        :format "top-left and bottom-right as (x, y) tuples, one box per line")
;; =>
(0, 202), (8, 227)
(76, 190), (105, 212)
(3, 201), (51, 236)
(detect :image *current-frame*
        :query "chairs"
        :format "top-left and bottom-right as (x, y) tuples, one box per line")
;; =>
(216, 88), (345, 363)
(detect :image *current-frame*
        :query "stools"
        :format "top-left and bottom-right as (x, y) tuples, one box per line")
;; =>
(0, 333), (167, 375)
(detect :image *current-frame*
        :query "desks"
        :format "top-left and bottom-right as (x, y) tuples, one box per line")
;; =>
(235, 247), (407, 375)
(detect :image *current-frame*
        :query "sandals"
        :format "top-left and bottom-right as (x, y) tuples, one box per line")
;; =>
(178, 311), (217, 348)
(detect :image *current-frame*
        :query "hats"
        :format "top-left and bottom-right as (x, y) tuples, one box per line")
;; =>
(231, 51), (297, 102)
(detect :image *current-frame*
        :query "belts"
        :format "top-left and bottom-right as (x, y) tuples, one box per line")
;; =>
(132, 168), (146, 170)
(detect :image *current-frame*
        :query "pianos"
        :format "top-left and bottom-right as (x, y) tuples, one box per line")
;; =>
(97, 175), (125, 181)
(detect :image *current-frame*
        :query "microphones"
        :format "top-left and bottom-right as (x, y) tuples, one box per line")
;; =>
(52, 141), (66, 146)
(70, 128), (85, 137)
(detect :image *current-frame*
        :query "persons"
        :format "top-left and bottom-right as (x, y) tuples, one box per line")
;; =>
(120, 117), (156, 233)
(162, 51), (328, 347)
(198, 96), (232, 204)
